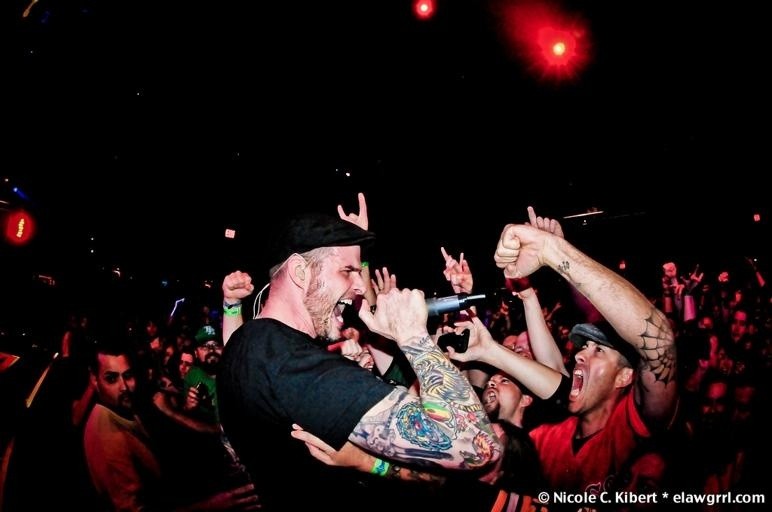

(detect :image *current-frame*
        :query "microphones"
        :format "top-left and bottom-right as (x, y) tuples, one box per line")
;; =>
(368, 289), (487, 318)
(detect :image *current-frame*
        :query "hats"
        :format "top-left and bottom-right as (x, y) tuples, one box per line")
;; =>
(259, 219), (376, 268)
(195, 326), (216, 346)
(568, 323), (640, 369)
